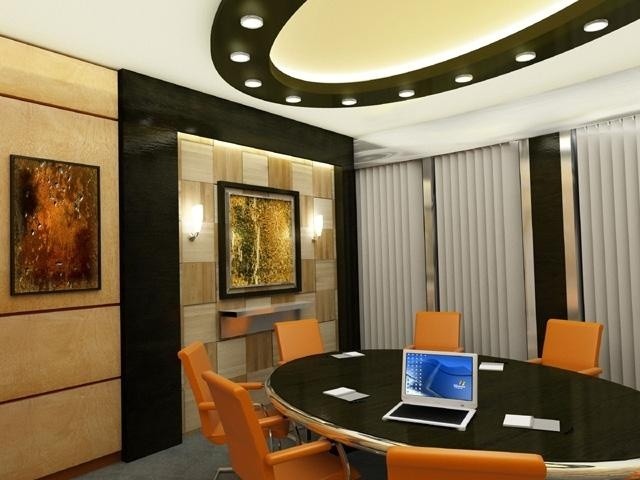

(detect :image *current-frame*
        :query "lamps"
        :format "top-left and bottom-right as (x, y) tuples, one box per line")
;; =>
(184, 204), (203, 241)
(310, 213), (324, 241)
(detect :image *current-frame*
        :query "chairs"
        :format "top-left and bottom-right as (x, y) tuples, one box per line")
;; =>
(382, 443), (548, 478)
(272, 315), (328, 367)
(178, 339), (290, 478)
(525, 316), (601, 377)
(404, 312), (467, 357)
(199, 367), (360, 478)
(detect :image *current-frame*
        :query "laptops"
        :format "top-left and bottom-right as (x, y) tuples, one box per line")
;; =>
(381, 348), (479, 432)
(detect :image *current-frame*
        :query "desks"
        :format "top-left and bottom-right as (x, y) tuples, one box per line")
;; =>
(264, 348), (640, 480)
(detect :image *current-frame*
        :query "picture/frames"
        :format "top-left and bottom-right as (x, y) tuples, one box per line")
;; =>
(10, 155), (102, 296)
(217, 180), (302, 301)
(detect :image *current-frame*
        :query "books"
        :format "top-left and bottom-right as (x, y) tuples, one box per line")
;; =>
(323, 386), (370, 401)
(479, 361), (504, 372)
(330, 351), (365, 359)
(503, 412), (560, 432)
